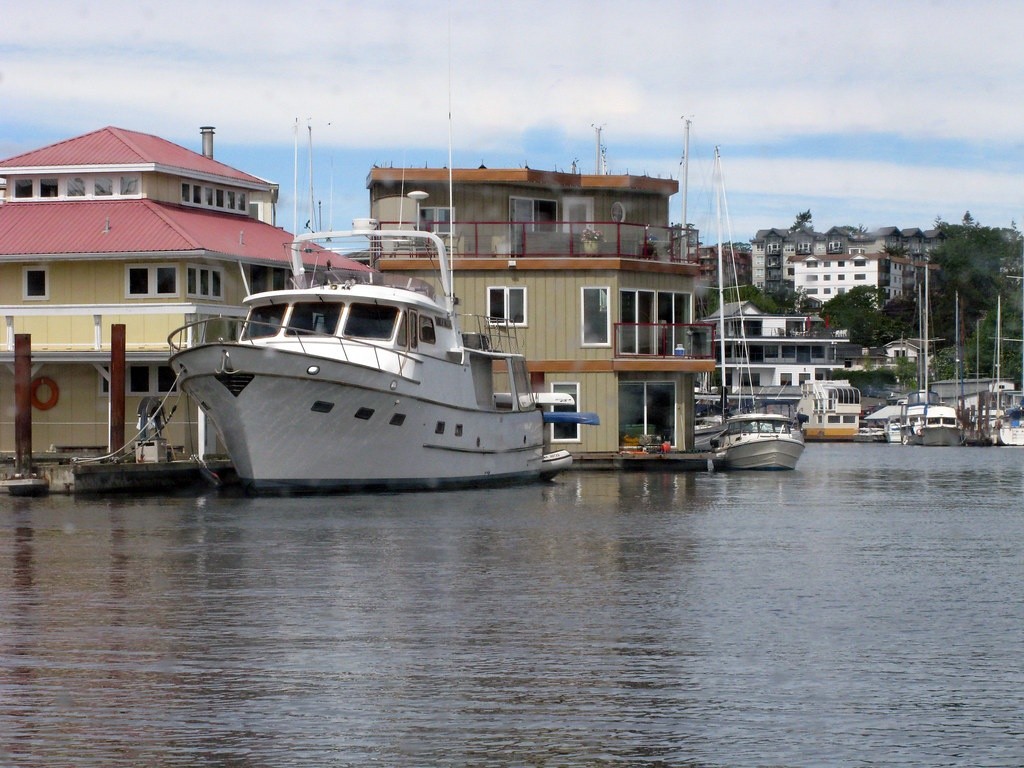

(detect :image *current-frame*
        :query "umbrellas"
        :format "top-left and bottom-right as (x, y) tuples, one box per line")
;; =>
(825, 314), (829, 329)
(806, 316), (810, 330)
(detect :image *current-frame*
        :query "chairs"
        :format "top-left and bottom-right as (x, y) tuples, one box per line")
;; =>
(389, 231), (463, 258)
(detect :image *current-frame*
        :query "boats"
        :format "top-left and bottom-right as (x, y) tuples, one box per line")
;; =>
(165, 110), (575, 492)
(794, 379), (884, 442)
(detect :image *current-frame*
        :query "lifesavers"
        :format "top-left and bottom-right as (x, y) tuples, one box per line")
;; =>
(32, 377), (59, 411)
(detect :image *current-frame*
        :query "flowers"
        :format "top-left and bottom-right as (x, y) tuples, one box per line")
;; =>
(583, 226), (604, 242)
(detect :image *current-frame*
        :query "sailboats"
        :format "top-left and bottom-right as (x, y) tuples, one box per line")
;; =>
(694, 145), (807, 470)
(885, 261), (1024, 447)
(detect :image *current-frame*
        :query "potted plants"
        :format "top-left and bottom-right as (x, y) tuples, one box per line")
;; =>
(638, 238), (657, 261)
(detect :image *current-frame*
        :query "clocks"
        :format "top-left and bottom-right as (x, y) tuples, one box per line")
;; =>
(610, 202), (626, 222)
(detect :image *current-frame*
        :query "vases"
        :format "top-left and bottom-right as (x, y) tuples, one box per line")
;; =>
(583, 240), (602, 256)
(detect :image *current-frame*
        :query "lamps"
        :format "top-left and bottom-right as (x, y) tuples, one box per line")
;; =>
(507, 260), (517, 269)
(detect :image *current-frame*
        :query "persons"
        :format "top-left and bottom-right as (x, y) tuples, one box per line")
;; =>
(910, 421), (916, 435)
